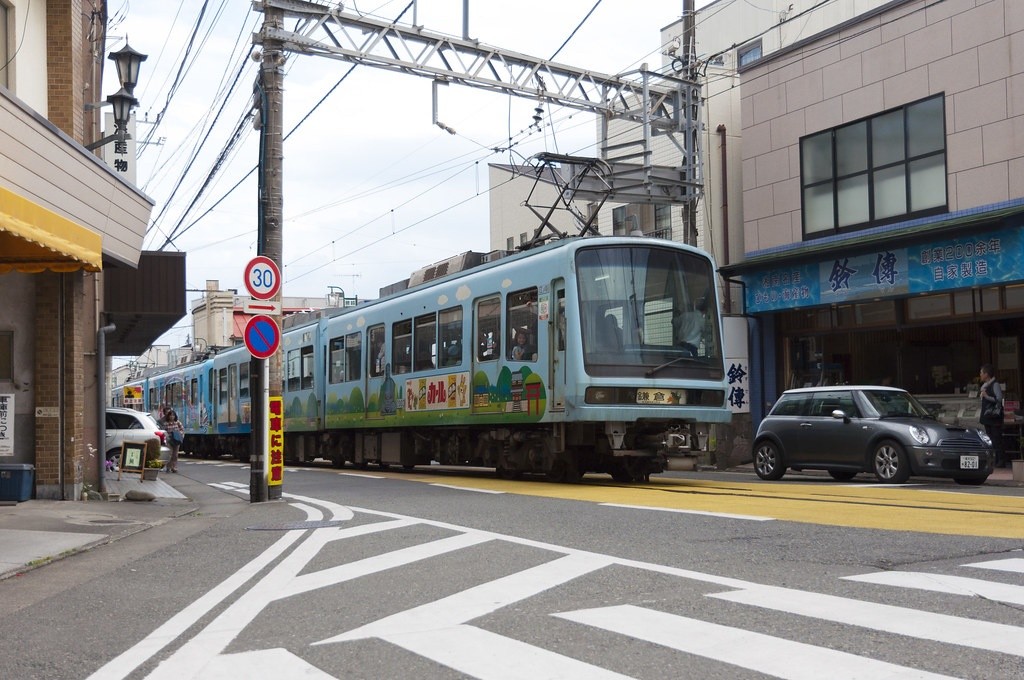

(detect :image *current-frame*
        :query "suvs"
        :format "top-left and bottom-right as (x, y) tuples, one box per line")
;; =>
(105, 408), (174, 472)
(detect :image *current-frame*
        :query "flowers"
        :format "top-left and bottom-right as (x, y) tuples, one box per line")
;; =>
(148, 458), (163, 469)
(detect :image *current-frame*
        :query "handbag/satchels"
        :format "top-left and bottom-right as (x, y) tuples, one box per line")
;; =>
(980, 396), (1004, 427)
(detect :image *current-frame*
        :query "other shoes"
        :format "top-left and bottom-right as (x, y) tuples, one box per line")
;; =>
(167, 463), (177, 473)
(993, 460), (1005, 468)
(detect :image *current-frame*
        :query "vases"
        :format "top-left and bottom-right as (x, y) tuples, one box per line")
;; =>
(143, 468), (159, 481)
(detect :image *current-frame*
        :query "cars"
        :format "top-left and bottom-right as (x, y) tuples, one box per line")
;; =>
(749, 385), (995, 486)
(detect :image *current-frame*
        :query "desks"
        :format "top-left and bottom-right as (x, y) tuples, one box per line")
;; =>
(1002, 417), (1024, 467)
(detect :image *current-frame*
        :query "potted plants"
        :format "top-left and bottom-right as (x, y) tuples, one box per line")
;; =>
(1011, 436), (1024, 481)
(145, 438), (160, 468)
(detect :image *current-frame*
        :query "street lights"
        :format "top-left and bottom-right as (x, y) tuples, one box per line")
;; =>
(193, 337), (208, 358)
(327, 285), (345, 310)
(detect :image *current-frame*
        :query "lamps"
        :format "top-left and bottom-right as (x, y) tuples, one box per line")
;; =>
(85, 79), (138, 151)
(83, 33), (149, 112)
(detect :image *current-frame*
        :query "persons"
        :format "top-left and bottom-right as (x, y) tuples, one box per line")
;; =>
(980, 364), (1005, 467)
(671, 299), (708, 358)
(377, 336), (386, 374)
(605, 314), (623, 350)
(159, 406), (171, 428)
(163, 410), (185, 472)
(510, 330), (537, 361)
(526, 302), (565, 351)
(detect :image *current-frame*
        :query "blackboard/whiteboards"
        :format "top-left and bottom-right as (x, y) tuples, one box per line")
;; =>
(121, 440), (147, 472)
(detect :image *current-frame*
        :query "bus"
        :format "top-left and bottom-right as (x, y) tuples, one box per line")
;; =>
(111, 234), (734, 483)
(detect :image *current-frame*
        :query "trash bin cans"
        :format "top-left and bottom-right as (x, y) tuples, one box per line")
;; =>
(0, 463), (34, 502)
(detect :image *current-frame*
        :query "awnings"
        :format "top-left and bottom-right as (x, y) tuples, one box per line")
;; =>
(0, 185), (105, 276)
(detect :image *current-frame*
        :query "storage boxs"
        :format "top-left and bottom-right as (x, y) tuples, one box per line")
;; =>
(0, 464), (36, 502)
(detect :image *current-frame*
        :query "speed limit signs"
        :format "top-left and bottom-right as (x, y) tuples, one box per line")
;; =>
(243, 257), (283, 300)
